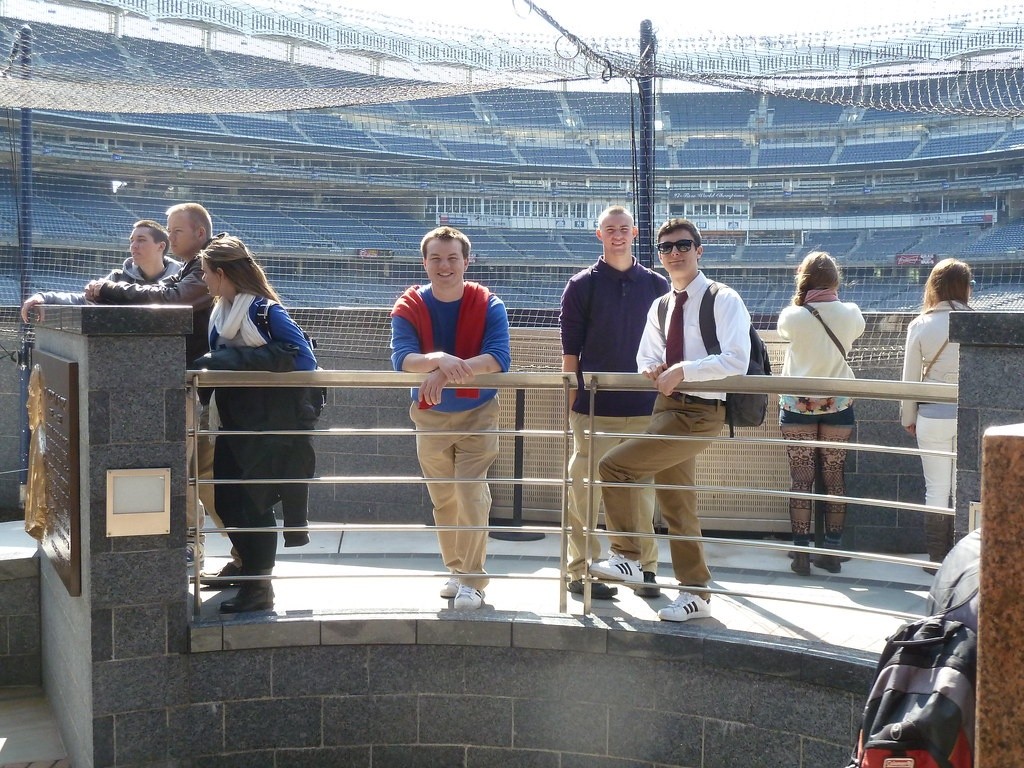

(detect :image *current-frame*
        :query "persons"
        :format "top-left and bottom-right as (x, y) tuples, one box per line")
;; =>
(189, 236), (318, 611)
(20, 218), (204, 566)
(899, 259), (974, 574)
(85, 204), (243, 588)
(559, 206), (669, 599)
(925, 528), (982, 635)
(587, 221), (752, 622)
(390, 227), (511, 609)
(777, 251), (867, 576)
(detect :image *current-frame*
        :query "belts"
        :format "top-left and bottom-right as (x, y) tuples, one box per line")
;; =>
(668, 392), (726, 406)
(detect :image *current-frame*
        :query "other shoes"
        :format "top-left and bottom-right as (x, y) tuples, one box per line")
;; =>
(634, 572), (660, 597)
(568, 578), (618, 599)
(209, 563), (241, 588)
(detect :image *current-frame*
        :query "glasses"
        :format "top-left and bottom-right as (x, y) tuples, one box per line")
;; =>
(656, 239), (699, 255)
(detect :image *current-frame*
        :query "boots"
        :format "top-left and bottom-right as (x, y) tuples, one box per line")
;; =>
(791, 544), (811, 575)
(922, 513), (949, 578)
(813, 541), (842, 573)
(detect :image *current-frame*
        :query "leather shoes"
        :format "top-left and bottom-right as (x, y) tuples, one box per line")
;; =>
(221, 584), (274, 612)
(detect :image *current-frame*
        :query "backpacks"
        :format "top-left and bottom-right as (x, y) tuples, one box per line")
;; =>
(255, 297), (327, 430)
(658, 281), (771, 427)
(842, 568), (980, 768)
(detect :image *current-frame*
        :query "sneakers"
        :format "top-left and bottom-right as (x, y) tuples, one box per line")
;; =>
(588, 550), (644, 584)
(657, 592), (712, 621)
(186, 544), (204, 567)
(454, 585), (483, 609)
(440, 577), (460, 597)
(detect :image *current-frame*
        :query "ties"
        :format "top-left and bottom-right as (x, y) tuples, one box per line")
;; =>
(666, 291), (688, 401)
